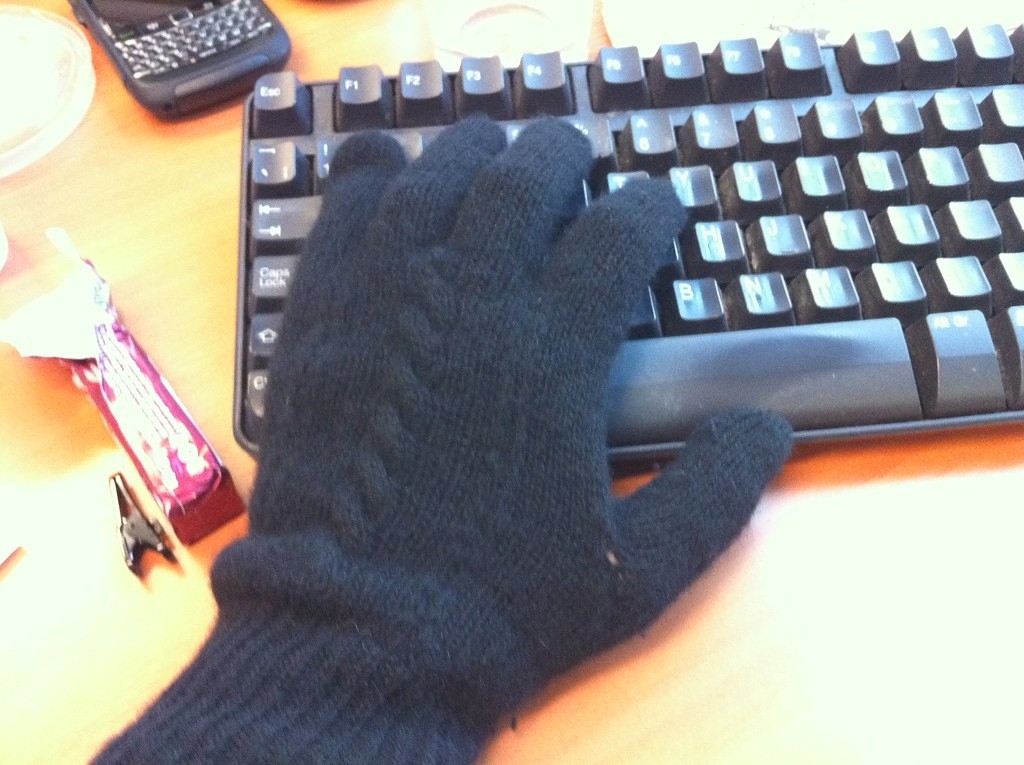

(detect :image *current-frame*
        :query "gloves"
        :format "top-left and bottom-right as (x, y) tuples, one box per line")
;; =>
(103, 115), (789, 765)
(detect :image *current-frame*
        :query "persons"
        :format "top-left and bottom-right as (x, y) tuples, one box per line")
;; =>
(89, 118), (795, 765)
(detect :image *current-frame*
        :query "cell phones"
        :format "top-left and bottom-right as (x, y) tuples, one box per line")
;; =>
(68, 0), (292, 118)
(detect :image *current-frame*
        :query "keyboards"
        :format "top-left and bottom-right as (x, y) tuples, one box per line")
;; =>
(233, 23), (1024, 472)
